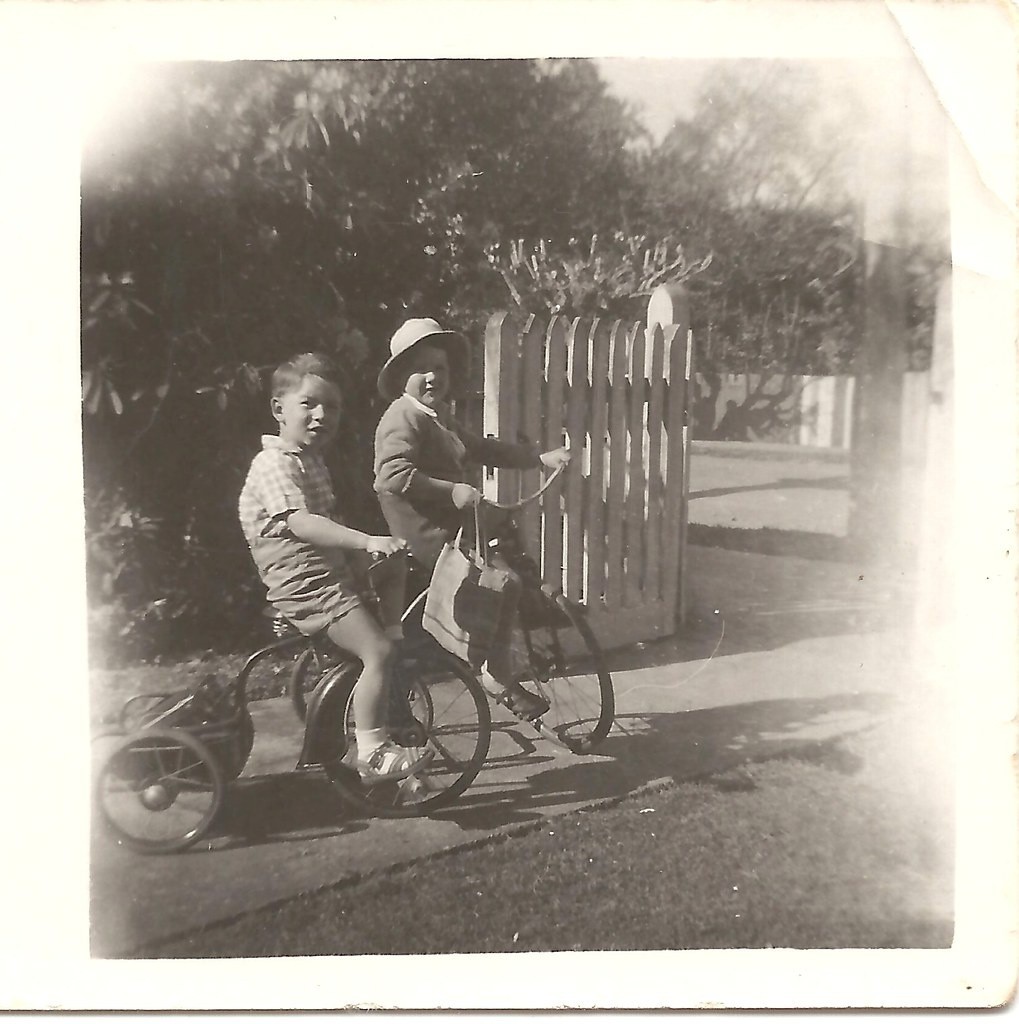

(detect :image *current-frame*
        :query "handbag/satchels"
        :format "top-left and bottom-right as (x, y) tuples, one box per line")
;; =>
(422, 487), (510, 669)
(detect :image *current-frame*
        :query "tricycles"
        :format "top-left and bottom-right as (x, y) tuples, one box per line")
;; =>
(92, 545), (493, 857)
(288, 426), (616, 755)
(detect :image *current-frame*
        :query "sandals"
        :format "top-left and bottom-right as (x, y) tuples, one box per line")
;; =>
(481, 676), (550, 721)
(350, 739), (436, 786)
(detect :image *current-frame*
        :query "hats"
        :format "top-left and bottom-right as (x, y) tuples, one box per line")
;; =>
(375, 317), (473, 401)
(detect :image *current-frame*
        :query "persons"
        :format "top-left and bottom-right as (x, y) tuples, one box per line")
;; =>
(372, 315), (576, 722)
(236, 351), (438, 787)
